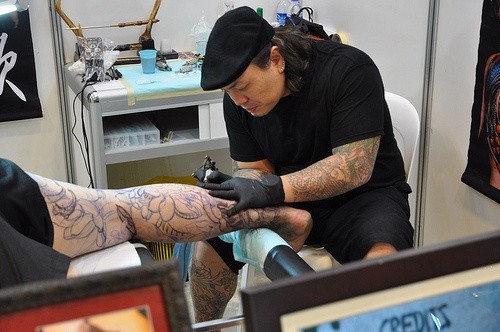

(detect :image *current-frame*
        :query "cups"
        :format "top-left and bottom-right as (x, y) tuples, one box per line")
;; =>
(139, 50), (157, 74)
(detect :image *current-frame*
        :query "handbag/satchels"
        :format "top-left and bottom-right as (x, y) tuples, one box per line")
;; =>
(290, 6), (328, 41)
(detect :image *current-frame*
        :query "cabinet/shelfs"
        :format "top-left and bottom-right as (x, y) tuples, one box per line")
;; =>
(64, 50), (229, 189)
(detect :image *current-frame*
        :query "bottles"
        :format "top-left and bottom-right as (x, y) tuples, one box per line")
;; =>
(257, 8), (263, 18)
(287, 0), (303, 27)
(276, 0), (287, 27)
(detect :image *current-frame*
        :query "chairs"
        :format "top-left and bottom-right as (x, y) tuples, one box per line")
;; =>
(238, 92), (421, 288)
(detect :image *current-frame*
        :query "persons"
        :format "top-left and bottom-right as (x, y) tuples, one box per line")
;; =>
(0, 155), (312, 279)
(189, 5), (414, 332)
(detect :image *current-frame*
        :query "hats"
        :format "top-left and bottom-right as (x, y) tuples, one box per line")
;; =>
(201, 6), (274, 91)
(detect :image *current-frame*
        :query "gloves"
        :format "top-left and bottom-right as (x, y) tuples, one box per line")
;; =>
(195, 169), (285, 217)
